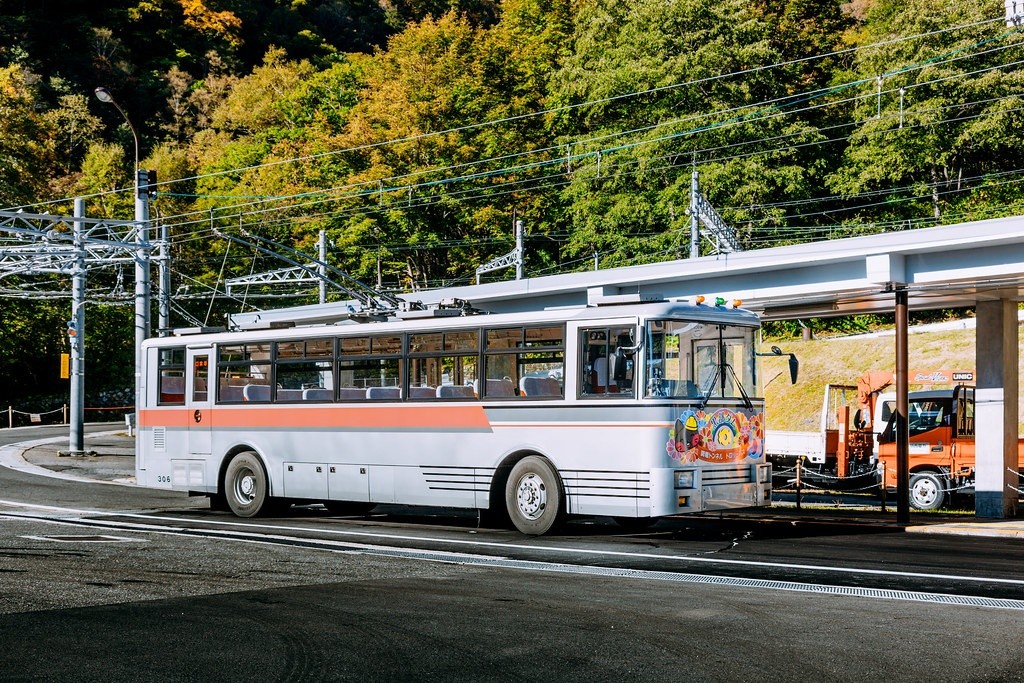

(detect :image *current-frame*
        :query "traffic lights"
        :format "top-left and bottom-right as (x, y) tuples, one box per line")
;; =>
(67, 320), (78, 338)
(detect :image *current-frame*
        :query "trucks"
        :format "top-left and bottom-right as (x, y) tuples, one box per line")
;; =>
(765, 382), (940, 494)
(853, 382), (1024, 518)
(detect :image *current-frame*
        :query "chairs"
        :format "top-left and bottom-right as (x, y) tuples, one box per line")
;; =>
(195, 377), (207, 401)
(161, 377), (185, 403)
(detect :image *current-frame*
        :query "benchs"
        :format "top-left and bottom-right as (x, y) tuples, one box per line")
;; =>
(366, 386), (401, 399)
(474, 376), (516, 399)
(340, 386), (370, 399)
(220, 386), (245, 401)
(302, 389), (333, 400)
(519, 377), (561, 396)
(243, 384), (271, 401)
(400, 385), (437, 398)
(436, 382), (474, 398)
(277, 390), (305, 400)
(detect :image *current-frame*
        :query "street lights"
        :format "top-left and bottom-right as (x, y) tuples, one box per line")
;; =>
(94, 86), (149, 389)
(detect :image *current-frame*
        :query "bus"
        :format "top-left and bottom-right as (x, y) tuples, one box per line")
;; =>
(134, 226), (799, 539)
